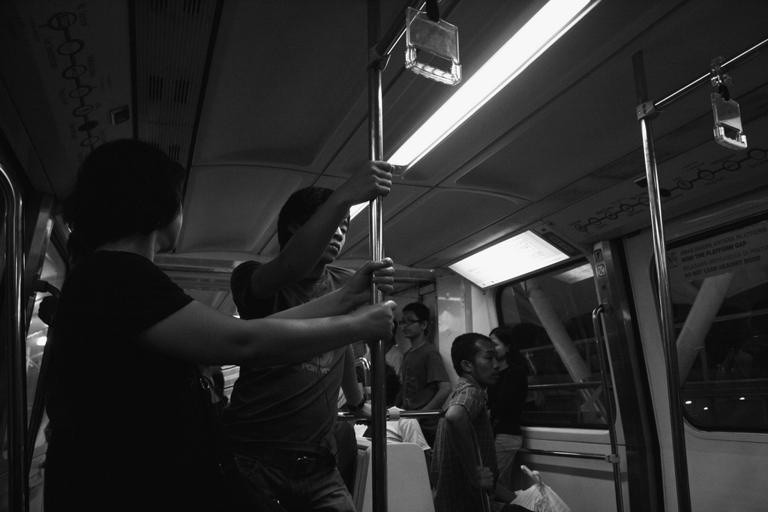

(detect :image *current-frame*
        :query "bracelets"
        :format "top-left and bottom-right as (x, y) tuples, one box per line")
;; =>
(345, 395), (368, 412)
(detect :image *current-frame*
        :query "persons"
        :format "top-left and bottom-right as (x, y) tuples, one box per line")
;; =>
(429, 330), (520, 511)
(39, 133), (403, 512)
(487, 326), (530, 495)
(391, 299), (450, 421)
(217, 154), (396, 511)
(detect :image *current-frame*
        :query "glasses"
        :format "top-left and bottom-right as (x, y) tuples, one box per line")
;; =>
(398, 317), (422, 326)
